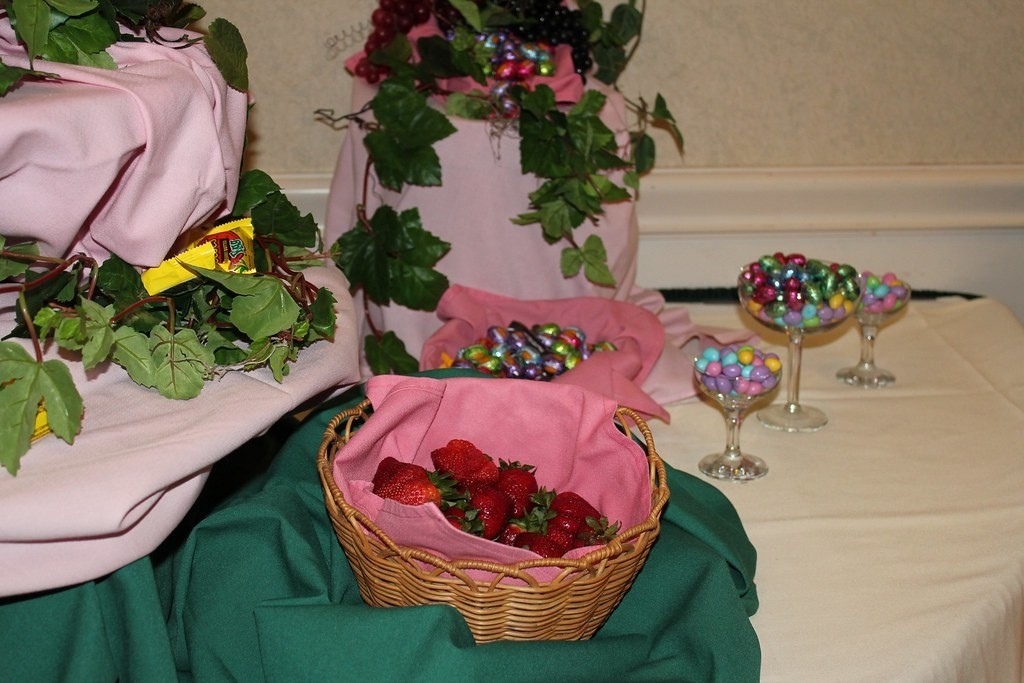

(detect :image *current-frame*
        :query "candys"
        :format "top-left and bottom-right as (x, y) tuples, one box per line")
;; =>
(452, 319), (620, 382)
(740, 252), (906, 329)
(695, 343), (781, 396)
(447, 28), (555, 113)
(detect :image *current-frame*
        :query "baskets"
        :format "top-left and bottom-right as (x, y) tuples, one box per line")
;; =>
(316, 402), (669, 647)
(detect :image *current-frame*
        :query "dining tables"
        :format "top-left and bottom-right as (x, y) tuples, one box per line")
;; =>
(644, 277), (1022, 683)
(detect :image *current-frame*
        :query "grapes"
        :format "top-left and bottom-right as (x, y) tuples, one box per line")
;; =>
(355, 0), (433, 83)
(511, 1), (593, 77)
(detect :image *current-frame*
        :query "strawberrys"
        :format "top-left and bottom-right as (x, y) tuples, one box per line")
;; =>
(373, 439), (621, 559)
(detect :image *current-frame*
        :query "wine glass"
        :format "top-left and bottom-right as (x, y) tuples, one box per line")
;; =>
(690, 353), (783, 482)
(834, 276), (911, 390)
(736, 257), (866, 433)
